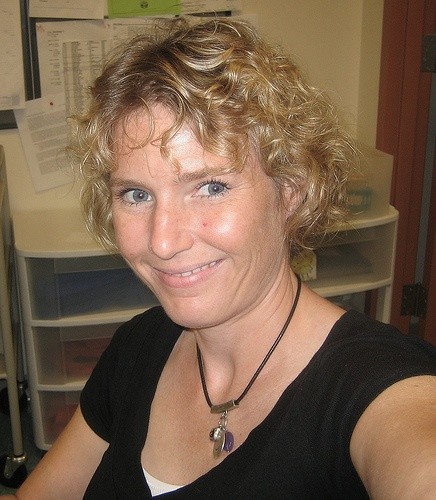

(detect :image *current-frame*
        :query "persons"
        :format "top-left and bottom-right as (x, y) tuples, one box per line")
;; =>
(193, 268), (303, 459)
(0, 15), (434, 500)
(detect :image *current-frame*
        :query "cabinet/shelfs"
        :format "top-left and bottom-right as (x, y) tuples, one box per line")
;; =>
(3, 131), (399, 455)
